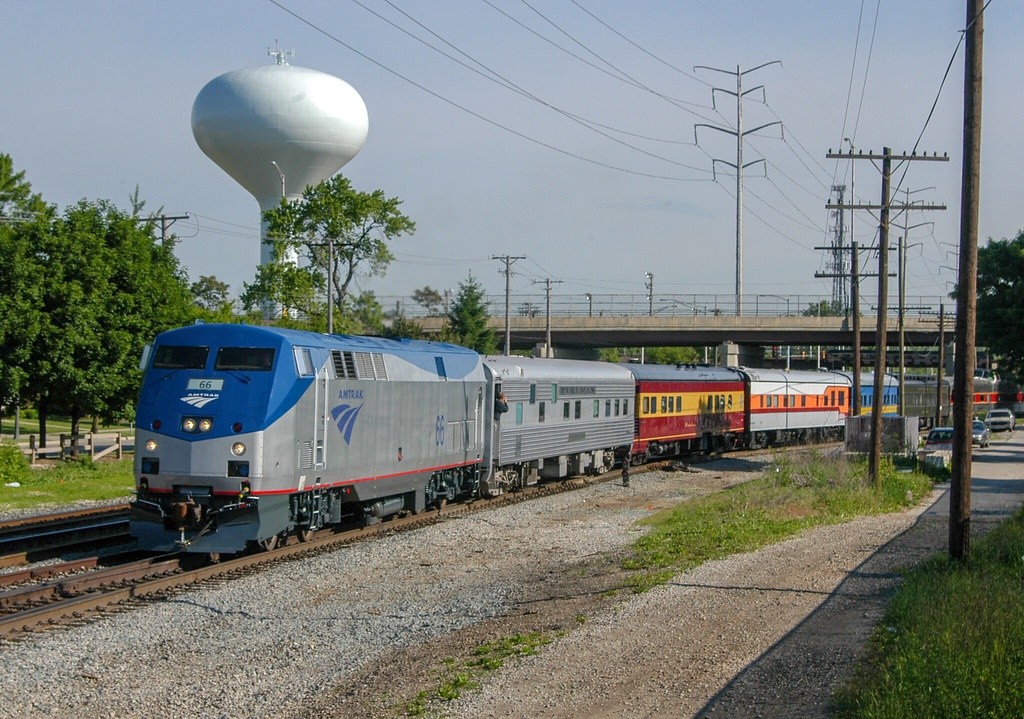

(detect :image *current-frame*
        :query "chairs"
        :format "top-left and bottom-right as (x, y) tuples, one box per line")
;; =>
(942, 435), (948, 439)
(933, 435), (940, 440)
(975, 424), (981, 428)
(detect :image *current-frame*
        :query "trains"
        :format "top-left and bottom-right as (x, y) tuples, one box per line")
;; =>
(131, 317), (1024, 563)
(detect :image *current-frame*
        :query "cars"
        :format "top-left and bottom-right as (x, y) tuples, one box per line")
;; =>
(971, 420), (990, 449)
(922, 426), (954, 452)
(983, 409), (1015, 433)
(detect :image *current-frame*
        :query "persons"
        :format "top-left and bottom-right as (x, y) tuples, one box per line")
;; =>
(494, 393), (509, 421)
